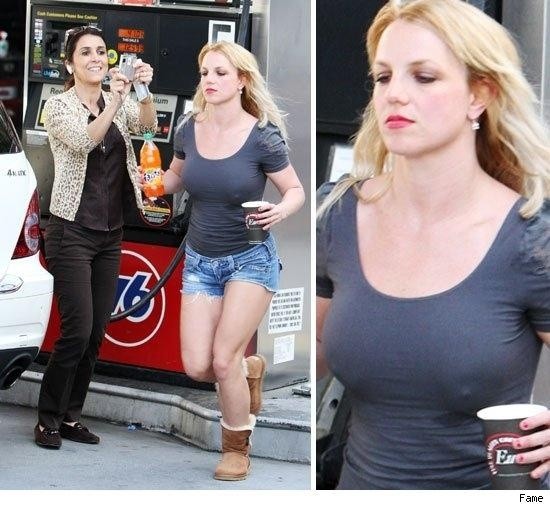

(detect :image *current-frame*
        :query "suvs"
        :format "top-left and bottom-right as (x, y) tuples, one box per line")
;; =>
(0, 99), (55, 390)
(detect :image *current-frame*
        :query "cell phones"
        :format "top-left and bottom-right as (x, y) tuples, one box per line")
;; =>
(119, 54), (136, 81)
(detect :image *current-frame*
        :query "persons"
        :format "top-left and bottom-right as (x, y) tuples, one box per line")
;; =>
(34, 26), (158, 448)
(316, 0), (550, 490)
(136, 41), (305, 481)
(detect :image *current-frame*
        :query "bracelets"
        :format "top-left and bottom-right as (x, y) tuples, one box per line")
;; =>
(137, 92), (154, 105)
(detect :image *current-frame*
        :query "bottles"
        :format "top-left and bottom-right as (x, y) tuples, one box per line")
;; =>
(140, 132), (164, 198)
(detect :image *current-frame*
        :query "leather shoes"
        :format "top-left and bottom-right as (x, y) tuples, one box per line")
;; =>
(34, 422), (99, 448)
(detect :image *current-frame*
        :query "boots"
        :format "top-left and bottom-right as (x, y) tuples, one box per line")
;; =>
(214, 414), (256, 482)
(241, 355), (267, 416)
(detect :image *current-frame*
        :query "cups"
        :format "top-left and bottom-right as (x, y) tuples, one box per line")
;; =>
(476, 404), (550, 490)
(240, 200), (270, 245)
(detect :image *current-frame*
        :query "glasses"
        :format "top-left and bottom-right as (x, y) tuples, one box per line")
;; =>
(64, 25), (102, 51)
